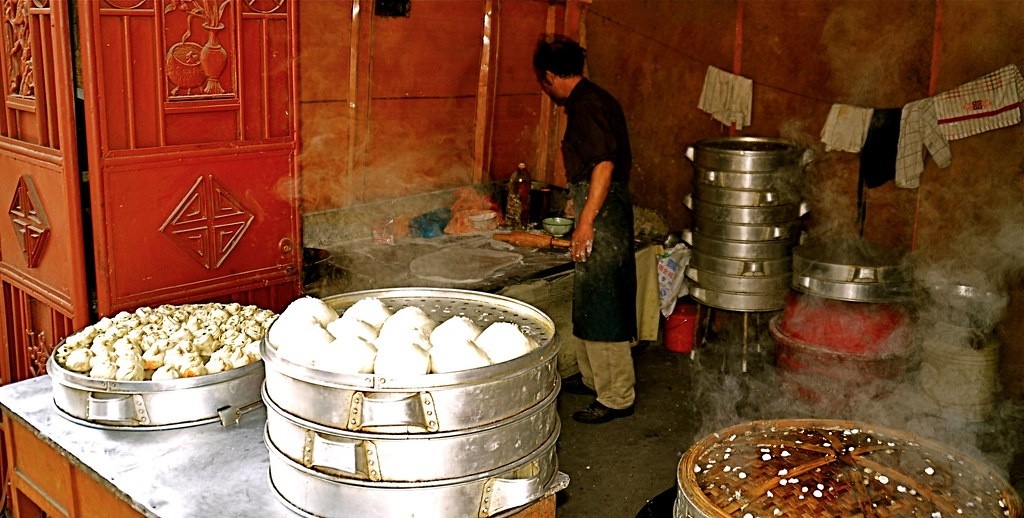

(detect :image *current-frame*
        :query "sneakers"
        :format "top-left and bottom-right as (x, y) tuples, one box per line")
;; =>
(573, 400), (634, 424)
(562, 379), (597, 395)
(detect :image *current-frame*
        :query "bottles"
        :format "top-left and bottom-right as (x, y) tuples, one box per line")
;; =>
(505, 163), (532, 224)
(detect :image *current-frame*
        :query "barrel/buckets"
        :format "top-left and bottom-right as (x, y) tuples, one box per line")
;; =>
(769, 297), (911, 419)
(917, 322), (1000, 422)
(665, 305), (699, 352)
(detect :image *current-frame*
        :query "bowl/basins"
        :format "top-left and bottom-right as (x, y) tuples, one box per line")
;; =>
(930, 283), (1008, 331)
(543, 217), (573, 236)
(303, 248), (334, 282)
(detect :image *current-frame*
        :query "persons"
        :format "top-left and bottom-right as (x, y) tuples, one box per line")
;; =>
(534, 32), (638, 424)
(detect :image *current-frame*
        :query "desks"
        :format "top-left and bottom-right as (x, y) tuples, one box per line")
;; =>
(0, 374), (570, 518)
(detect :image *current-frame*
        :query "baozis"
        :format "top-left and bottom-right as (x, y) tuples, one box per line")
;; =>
(58, 303), (280, 383)
